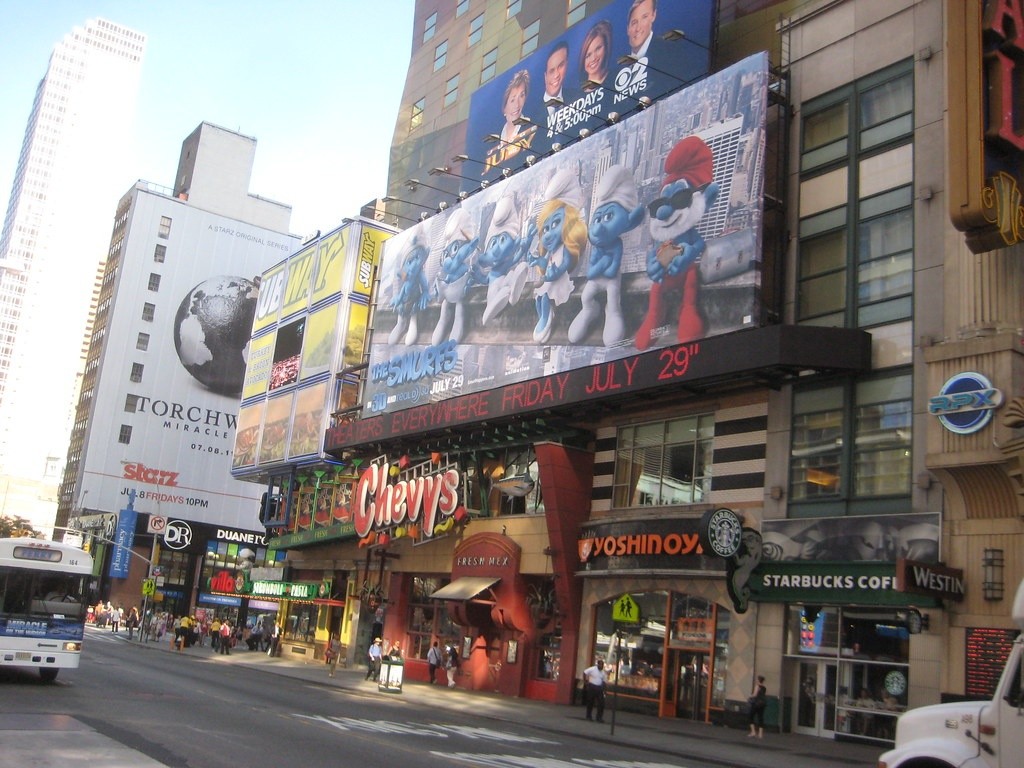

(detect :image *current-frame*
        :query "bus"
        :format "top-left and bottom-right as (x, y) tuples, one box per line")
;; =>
(0, 536), (95, 681)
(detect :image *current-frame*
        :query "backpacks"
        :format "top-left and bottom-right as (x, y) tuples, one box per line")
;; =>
(367, 644), (380, 657)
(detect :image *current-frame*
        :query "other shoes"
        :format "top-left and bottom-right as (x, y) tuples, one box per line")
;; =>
(597, 717), (604, 722)
(586, 717), (594, 721)
(747, 734), (756, 737)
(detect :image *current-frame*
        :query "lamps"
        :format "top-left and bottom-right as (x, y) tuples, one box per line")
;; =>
(482, 132), (546, 157)
(662, 28), (735, 63)
(428, 167), (490, 187)
(361, 206), (419, 223)
(452, 154), (515, 173)
(381, 196), (438, 212)
(616, 53), (691, 84)
(579, 79), (651, 108)
(404, 178), (465, 201)
(513, 117), (577, 140)
(543, 99), (613, 125)
(981, 548), (1005, 601)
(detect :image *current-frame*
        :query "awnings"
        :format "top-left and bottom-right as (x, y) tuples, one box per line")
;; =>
(430, 576), (502, 603)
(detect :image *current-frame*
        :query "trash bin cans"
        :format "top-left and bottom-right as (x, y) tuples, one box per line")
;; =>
(378, 655), (405, 694)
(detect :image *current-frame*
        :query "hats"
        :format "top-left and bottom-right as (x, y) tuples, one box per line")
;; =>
(373, 638), (382, 642)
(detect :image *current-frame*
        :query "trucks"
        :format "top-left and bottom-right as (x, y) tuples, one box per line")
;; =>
(875, 574), (1024, 768)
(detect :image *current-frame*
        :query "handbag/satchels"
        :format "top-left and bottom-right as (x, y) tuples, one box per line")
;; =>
(437, 659), (440, 669)
(751, 700), (766, 711)
(446, 661), (452, 669)
(326, 650), (332, 658)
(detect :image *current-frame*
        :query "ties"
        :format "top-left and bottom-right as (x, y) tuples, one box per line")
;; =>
(555, 98), (561, 113)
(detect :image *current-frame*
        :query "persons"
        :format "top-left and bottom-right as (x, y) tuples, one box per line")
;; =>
(365, 638), (385, 682)
(803, 677), (898, 746)
(584, 661), (610, 723)
(577, 18), (619, 107)
(44, 580), (78, 603)
(326, 632), (341, 677)
(444, 647), (459, 690)
(86, 600), (284, 658)
(543, 657), (553, 674)
(746, 675), (767, 738)
(622, 0), (707, 101)
(427, 642), (444, 686)
(540, 40), (587, 123)
(387, 641), (401, 661)
(482, 69), (539, 163)
